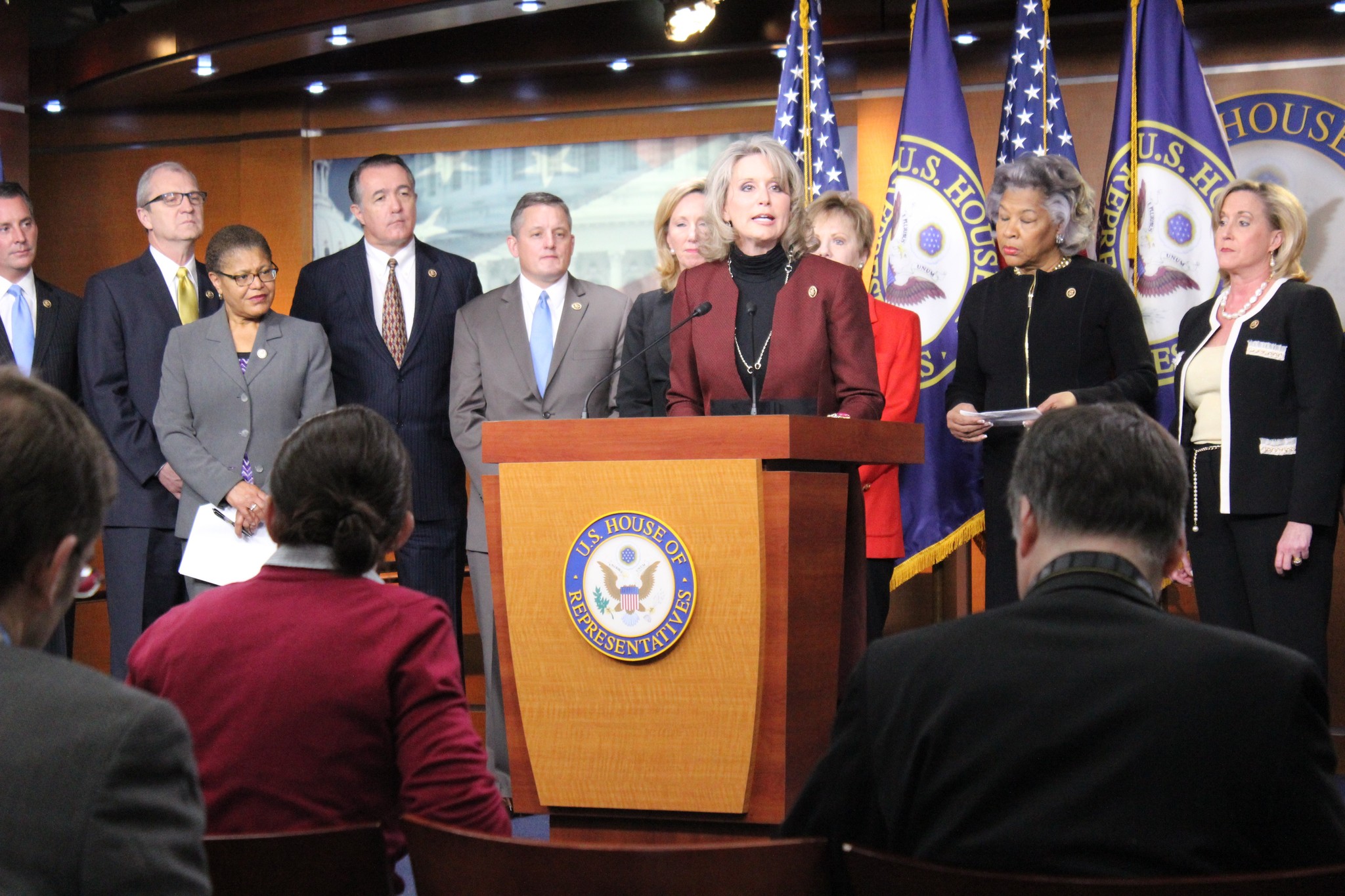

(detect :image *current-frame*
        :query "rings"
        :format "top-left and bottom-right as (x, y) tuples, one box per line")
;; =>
(250, 504), (257, 510)
(1293, 557), (1302, 564)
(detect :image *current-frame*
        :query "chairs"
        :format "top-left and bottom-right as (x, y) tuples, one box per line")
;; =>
(202, 814), (1345, 896)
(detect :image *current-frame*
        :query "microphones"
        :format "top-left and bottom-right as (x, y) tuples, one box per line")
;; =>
(580, 302), (712, 418)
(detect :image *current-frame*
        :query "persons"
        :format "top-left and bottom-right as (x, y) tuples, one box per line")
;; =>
(288, 149), (723, 824)
(0, 178), (90, 665)
(775, 403), (1345, 895)
(0, 359), (212, 896)
(792, 188), (922, 649)
(942, 154), (1160, 626)
(150, 222), (339, 602)
(71, 162), (221, 684)
(127, 404), (518, 896)
(662, 134), (884, 720)
(1163, 179), (1345, 697)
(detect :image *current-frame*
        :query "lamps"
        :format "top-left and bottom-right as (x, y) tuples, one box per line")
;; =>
(661, 0), (718, 43)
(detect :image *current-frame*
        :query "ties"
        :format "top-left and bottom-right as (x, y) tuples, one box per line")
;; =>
(172, 266), (200, 330)
(529, 290), (554, 404)
(7, 283), (36, 389)
(381, 258), (410, 374)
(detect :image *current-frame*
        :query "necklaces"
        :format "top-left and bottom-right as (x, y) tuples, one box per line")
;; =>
(1011, 255), (1075, 275)
(721, 245), (796, 378)
(1220, 271), (1276, 320)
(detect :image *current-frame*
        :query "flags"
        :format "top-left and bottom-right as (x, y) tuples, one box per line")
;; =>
(773, 0), (848, 193)
(1094, 0), (1241, 445)
(867, 0), (991, 596)
(991, 0), (1079, 188)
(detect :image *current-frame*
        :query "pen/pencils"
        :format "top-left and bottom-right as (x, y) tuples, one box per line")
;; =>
(211, 508), (254, 540)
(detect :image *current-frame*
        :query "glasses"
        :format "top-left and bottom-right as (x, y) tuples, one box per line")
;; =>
(209, 260), (279, 288)
(47, 543), (104, 601)
(138, 190), (209, 208)
